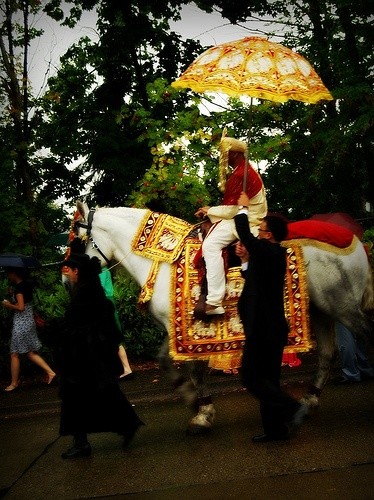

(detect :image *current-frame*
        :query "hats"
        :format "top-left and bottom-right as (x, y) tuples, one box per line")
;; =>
(214, 127), (248, 192)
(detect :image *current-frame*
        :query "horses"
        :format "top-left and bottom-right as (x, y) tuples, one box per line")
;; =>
(61, 192), (374, 438)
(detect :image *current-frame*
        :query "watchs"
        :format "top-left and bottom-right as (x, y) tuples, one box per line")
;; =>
(238, 205), (249, 209)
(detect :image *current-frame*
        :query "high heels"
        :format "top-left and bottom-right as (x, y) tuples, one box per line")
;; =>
(3, 382), (20, 394)
(46, 373), (58, 386)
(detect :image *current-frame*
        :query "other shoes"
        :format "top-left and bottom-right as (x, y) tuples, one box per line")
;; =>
(286, 406), (310, 428)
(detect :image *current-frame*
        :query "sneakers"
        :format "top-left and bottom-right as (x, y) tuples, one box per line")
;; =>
(205, 306), (225, 315)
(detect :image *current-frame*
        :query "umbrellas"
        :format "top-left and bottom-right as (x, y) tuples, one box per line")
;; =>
(171, 35), (335, 192)
(0, 254), (42, 268)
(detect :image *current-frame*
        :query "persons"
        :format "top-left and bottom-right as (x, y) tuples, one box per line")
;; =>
(0, 267), (60, 394)
(231, 191), (307, 443)
(32, 250), (146, 460)
(193, 136), (268, 316)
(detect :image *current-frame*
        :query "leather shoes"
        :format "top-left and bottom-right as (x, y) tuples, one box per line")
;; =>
(60, 443), (91, 459)
(116, 372), (135, 382)
(251, 429), (291, 443)
(333, 376), (359, 385)
(122, 421), (142, 450)
